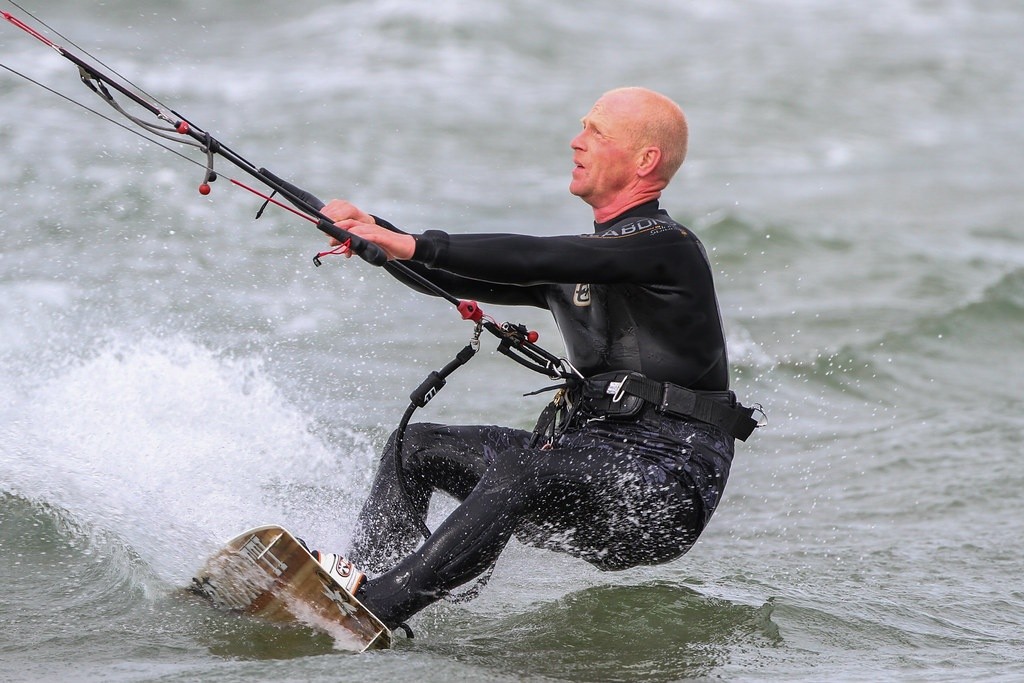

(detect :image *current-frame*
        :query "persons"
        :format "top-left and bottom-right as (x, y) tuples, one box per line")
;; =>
(294, 85), (768, 632)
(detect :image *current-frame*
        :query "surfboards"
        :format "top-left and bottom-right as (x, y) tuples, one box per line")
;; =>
(189, 523), (396, 652)
